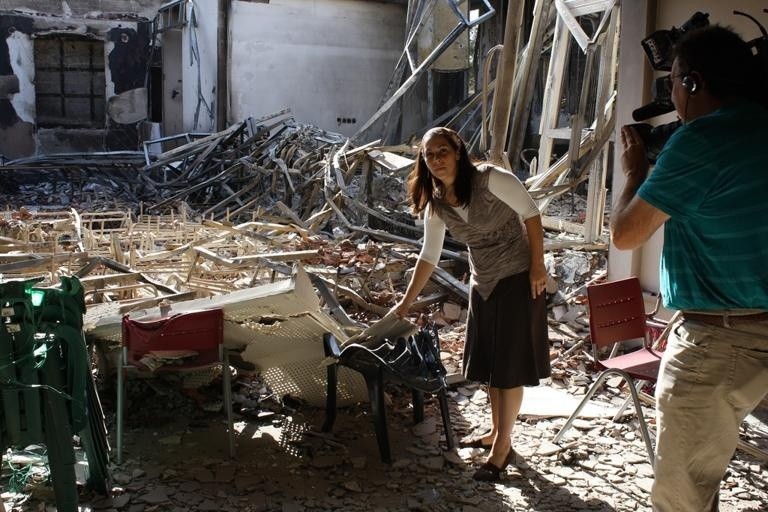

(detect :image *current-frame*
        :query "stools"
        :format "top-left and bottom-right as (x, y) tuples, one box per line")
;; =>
(321, 323), (454, 463)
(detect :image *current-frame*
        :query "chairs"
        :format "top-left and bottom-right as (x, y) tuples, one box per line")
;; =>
(552, 275), (661, 474)
(116, 305), (236, 466)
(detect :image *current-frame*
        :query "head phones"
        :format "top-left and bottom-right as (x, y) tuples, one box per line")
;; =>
(681, 49), (700, 95)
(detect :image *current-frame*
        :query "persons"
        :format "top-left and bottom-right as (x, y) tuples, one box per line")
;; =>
(607, 24), (768, 512)
(375, 126), (551, 482)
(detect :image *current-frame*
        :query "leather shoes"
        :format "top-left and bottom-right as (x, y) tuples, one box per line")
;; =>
(459, 435), (493, 450)
(472, 445), (516, 481)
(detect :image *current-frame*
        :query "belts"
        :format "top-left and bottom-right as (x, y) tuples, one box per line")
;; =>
(679, 312), (768, 326)
